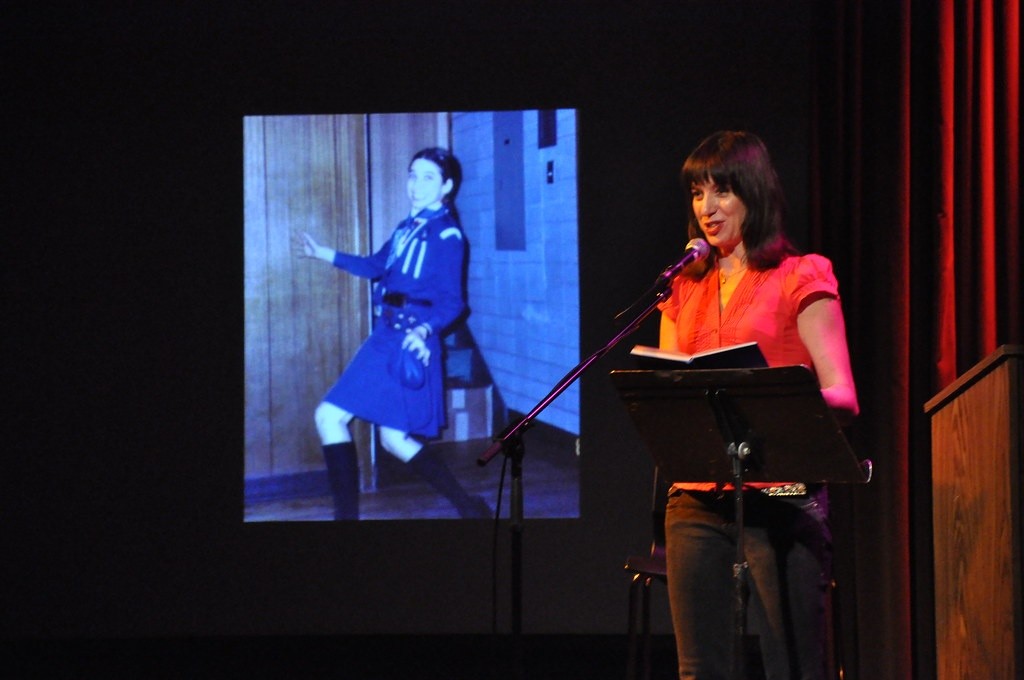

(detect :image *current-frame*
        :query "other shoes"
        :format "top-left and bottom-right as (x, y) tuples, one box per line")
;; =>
(462, 495), (493, 518)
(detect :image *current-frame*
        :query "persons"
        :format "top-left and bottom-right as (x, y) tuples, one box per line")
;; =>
(657, 130), (860, 680)
(290, 146), (493, 520)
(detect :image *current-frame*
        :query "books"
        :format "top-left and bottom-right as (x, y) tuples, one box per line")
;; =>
(630, 342), (767, 369)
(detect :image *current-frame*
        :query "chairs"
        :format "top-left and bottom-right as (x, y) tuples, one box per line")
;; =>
(622, 462), (750, 680)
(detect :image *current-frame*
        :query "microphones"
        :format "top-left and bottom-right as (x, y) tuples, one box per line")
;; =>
(655, 238), (710, 287)
